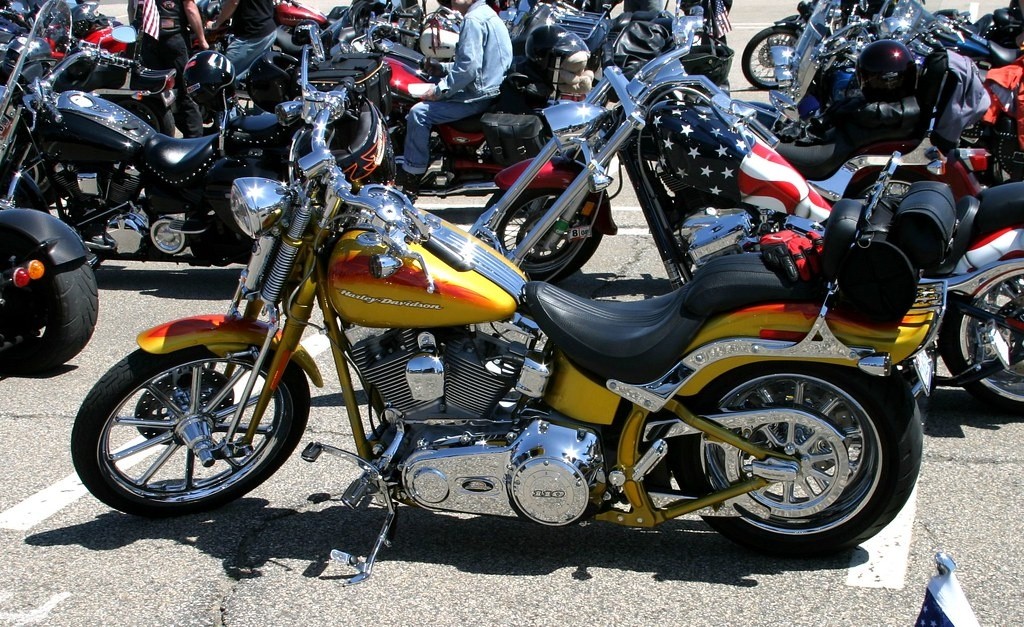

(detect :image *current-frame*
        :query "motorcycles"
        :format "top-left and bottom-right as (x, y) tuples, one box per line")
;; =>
(0, 0), (1024, 581)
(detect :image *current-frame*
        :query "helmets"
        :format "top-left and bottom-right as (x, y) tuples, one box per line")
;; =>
(245, 51), (299, 114)
(526, 25), (590, 73)
(678, 45), (735, 87)
(3, 33), (51, 75)
(294, 90), (397, 194)
(291, 19), (323, 45)
(855, 39), (917, 101)
(72, 2), (110, 38)
(184, 51), (237, 105)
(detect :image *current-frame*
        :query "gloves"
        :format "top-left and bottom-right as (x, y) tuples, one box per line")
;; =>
(760, 227), (827, 282)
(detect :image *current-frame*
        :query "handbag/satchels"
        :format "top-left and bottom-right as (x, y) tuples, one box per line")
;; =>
(917, 48), (991, 157)
(491, 58), (547, 114)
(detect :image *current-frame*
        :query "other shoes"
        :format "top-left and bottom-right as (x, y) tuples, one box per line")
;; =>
(393, 170), (420, 196)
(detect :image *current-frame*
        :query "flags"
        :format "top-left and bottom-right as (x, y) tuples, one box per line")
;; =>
(138, 0), (163, 42)
(714, 0), (733, 38)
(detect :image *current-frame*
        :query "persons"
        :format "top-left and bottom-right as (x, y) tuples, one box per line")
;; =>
(389, 0), (512, 185)
(678, 0), (733, 47)
(202, 0), (279, 80)
(126, 0), (211, 139)
(621, 0), (666, 13)
